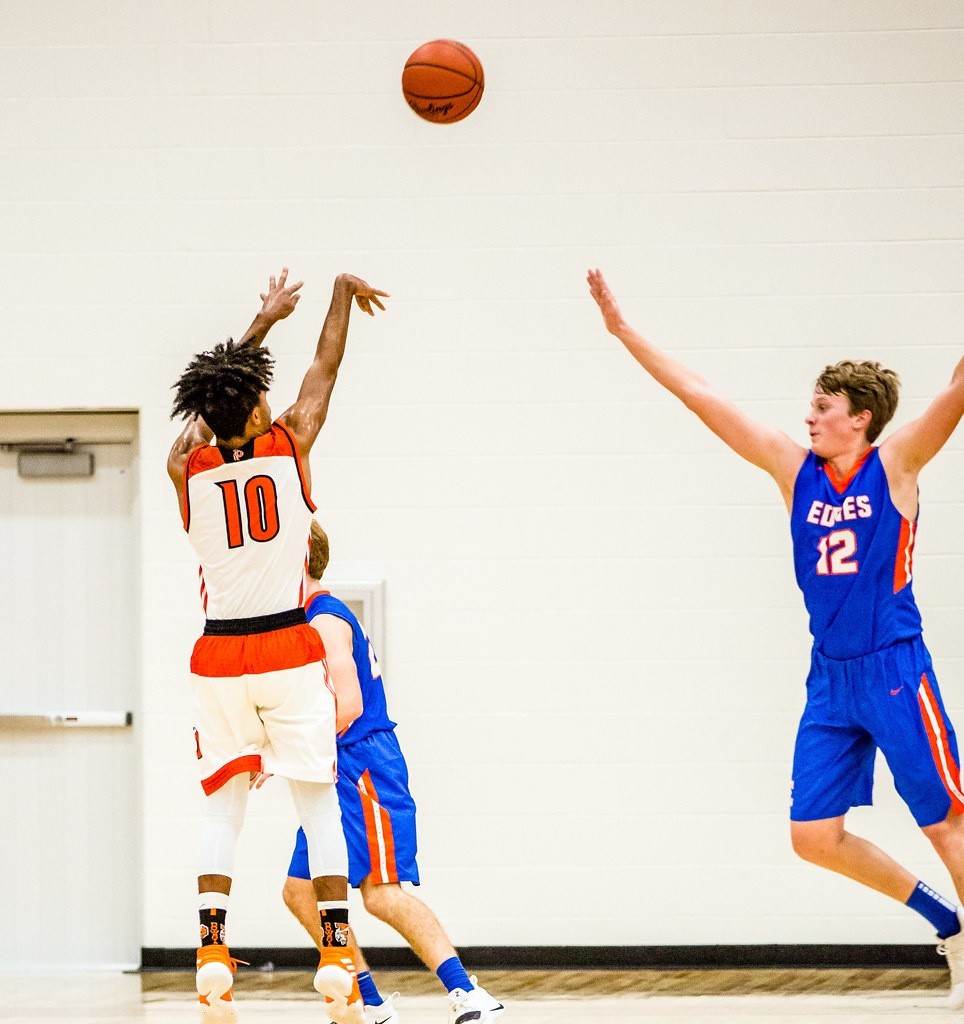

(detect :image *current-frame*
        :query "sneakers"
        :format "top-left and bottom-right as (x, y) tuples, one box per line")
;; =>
(313, 946), (363, 1024)
(196, 945), (250, 1024)
(935, 905), (964, 1009)
(450, 974), (505, 1024)
(364, 990), (400, 1024)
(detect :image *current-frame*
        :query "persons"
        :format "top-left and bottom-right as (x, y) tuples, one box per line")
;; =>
(249, 519), (505, 1024)
(168, 266), (390, 1024)
(587, 268), (964, 1010)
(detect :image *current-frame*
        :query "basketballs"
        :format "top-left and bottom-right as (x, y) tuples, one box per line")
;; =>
(400, 37), (486, 126)
